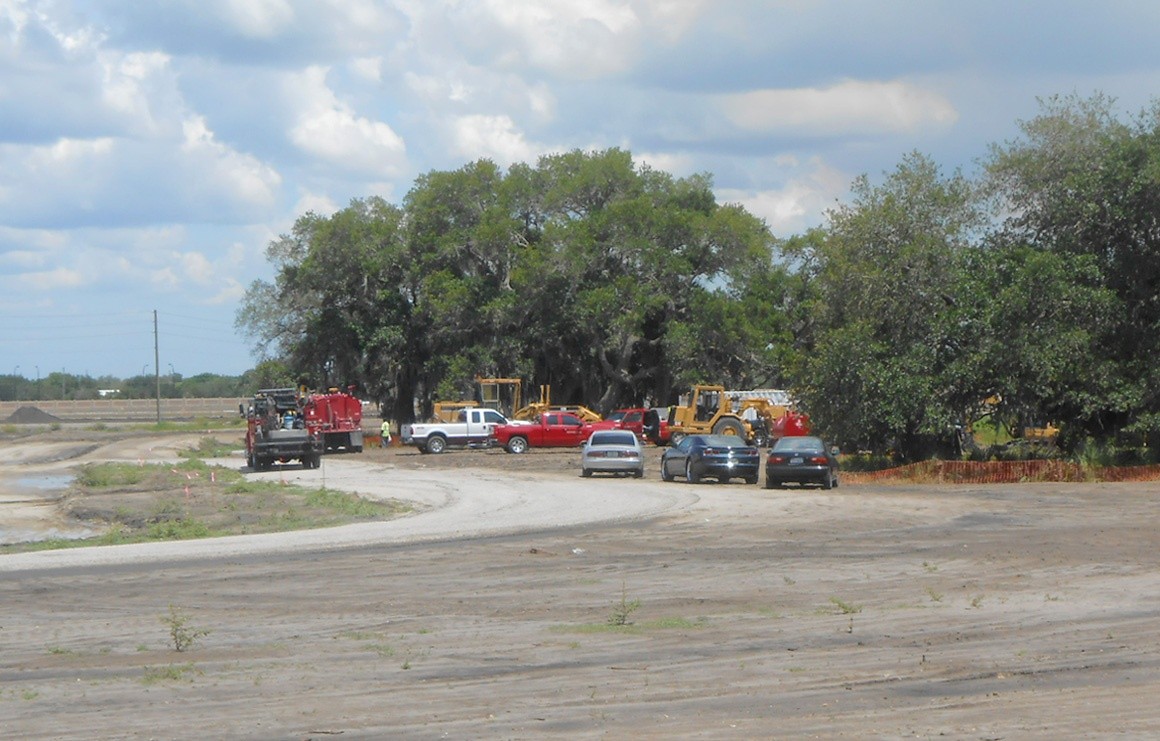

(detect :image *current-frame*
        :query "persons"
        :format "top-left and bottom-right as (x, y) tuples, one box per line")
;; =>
(380, 417), (390, 448)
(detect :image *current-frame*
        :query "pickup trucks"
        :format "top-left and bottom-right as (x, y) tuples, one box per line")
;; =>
(493, 412), (604, 454)
(605, 409), (684, 447)
(400, 407), (531, 453)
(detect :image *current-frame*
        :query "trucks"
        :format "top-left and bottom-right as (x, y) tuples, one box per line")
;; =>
(240, 386), (363, 472)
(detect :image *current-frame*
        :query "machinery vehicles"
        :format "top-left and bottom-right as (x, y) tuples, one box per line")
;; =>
(664, 384), (788, 447)
(433, 378), (604, 423)
(957, 392), (1063, 448)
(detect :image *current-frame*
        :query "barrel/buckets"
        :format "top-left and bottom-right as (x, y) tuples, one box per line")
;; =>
(284, 417), (294, 429)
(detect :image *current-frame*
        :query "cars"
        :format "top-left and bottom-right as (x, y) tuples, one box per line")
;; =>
(660, 434), (761, 485)
(578, 430), (647, 478)
(765, 435), (840, 489)
